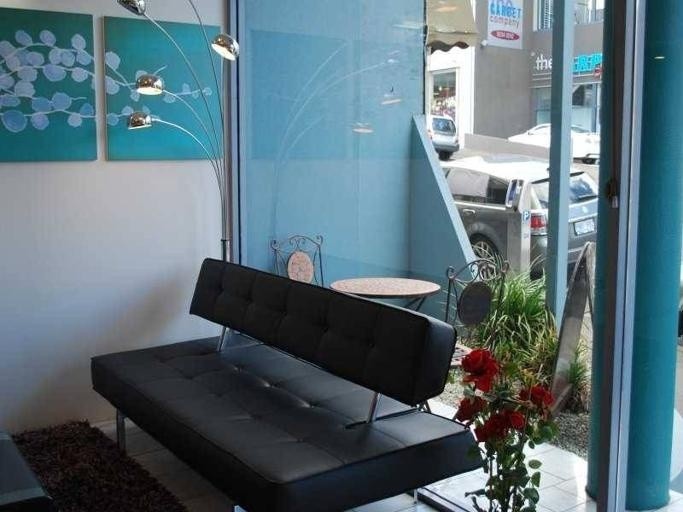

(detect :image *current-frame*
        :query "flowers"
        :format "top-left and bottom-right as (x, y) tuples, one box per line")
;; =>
(455, 350), (556, 511)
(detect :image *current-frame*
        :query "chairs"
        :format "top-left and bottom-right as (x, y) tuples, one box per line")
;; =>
(437, 259), (514, 367)
(275, 235), (327, 289)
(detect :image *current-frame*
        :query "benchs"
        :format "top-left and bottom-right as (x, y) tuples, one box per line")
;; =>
(92, 255), (489, 512)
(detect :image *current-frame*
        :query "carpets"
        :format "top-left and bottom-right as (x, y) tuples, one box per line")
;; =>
(3, 419), (186, 511)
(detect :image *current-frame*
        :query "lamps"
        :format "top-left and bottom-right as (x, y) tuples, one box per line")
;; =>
(119, 1), (238, 258)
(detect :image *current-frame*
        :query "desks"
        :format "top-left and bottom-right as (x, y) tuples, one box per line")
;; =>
(333, 276), (443, 418)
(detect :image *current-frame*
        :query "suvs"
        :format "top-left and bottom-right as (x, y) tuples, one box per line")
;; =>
(507, 122), (599, 165)
(423, 113), (459, 160)
(431, 157), (598, 285)
